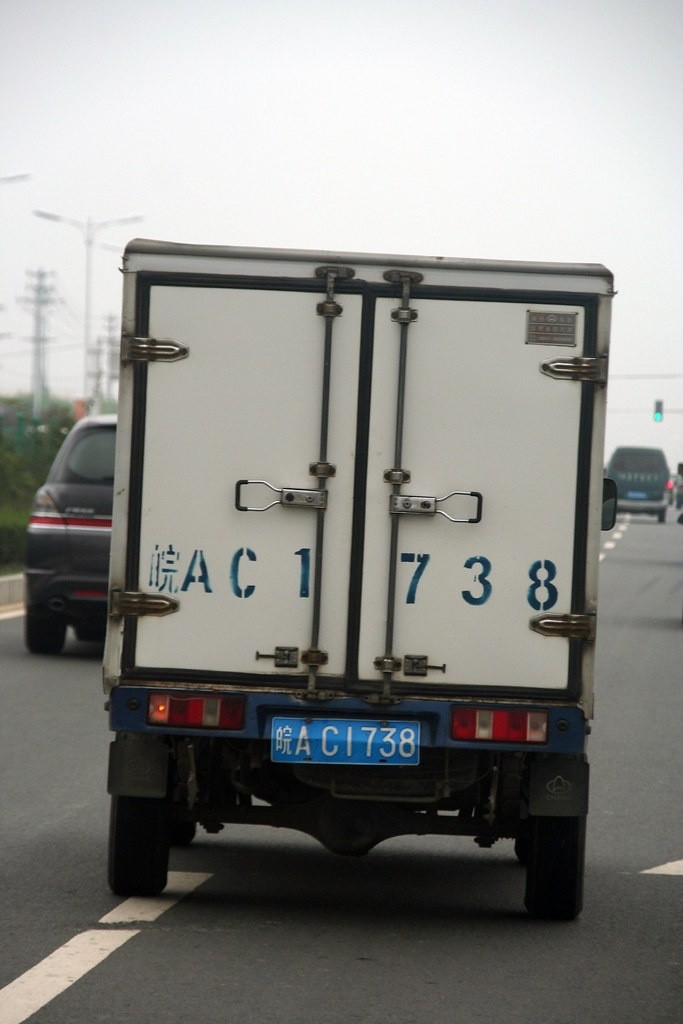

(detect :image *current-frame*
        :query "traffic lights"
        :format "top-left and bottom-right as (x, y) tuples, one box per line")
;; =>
(653, 401), (664, 422)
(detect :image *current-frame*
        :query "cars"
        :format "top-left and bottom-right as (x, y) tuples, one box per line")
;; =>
(20, 414), (119, 658)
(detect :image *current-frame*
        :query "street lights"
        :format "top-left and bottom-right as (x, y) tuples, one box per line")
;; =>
(36, 207), (150, 395)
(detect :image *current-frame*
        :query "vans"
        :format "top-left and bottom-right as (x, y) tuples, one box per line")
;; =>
(603, 446), (672, 523)
(673, 463), (683, 508)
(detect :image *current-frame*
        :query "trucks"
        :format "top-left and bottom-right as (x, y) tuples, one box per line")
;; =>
(100, 234), (621, 927)
